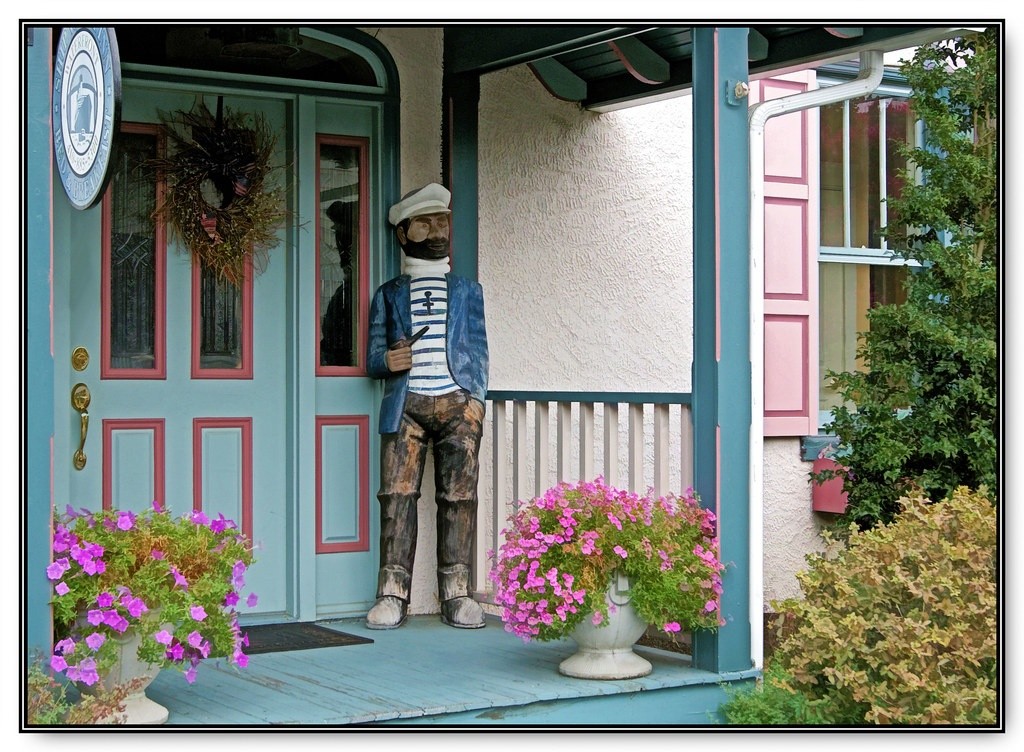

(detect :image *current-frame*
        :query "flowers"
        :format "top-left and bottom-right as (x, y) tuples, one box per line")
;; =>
(479, 469), (727, 646)
(45, 499), (258, 685)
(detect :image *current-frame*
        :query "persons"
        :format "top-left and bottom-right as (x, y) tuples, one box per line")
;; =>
(365, 182), (490, 628)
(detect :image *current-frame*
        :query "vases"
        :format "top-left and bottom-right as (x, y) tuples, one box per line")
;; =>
(558, 565), (655, 679)
(62, 610), (170, 726)
